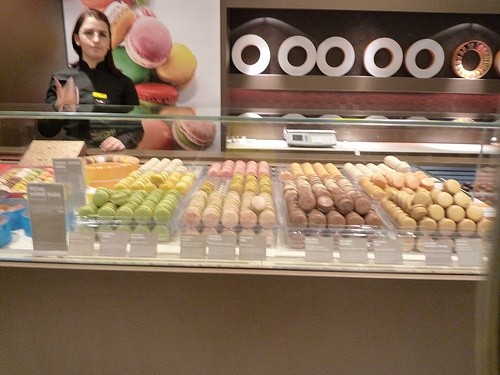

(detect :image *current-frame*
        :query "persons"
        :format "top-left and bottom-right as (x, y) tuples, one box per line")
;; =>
(38, 7), (145, 152)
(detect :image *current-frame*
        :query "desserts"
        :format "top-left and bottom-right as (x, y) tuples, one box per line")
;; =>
(79, 154), (197, 242)
(182, 160), (277, 245)
(342, 154), (494, 252)
(81, 0), (217, 151)
(280, 162), (383, 245)
(2, 166), (56, 193)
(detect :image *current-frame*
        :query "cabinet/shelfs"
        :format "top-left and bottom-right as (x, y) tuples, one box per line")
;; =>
(1, 110), (500, 375)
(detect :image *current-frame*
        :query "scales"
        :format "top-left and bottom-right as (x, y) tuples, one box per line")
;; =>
(282, 123), (338, 147)
